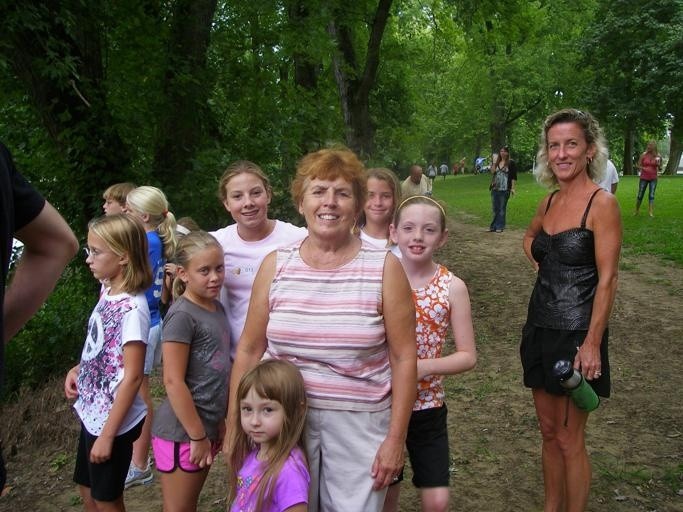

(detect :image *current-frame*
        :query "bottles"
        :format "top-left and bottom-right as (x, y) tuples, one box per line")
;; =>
(552, 359), (600, 412)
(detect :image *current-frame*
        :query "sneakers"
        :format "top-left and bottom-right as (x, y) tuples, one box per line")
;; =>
(496, 229), (503, 233)
(486, 229), (495, 232)
(129, 455), (154, 470)
(124, 464), (153, 491)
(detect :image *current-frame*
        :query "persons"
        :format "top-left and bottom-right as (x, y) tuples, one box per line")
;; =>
(632, 142), (662, 217)
(103, 183), (134, 216)
(594, 157), (619, 194)
(125, 186), (178, 489)
(0, 139), (80, 498)
(381, 195), (479, 511)
(522, 110), (622, 510)
(484, 148), (519, 232)
(65, 216), (150, 511)
(149, 232), (230, 512)
(352, 168), (405, 265)
(227, 360), (312, 511)
(207, 160), (308, 372)
(223, 143), (416, 511)
(401, 156), (483, 202)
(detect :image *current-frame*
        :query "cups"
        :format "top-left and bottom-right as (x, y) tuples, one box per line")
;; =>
(655, 157), (660, 165)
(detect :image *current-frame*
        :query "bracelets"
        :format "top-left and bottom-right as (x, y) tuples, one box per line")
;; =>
(189, 432), (207, 442)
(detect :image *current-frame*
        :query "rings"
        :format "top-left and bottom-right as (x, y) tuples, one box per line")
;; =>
(594, 369), (601, 376)
(391, 476), (400, 482)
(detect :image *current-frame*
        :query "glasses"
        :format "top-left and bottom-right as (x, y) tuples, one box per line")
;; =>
(83, 247), (104, 256)
